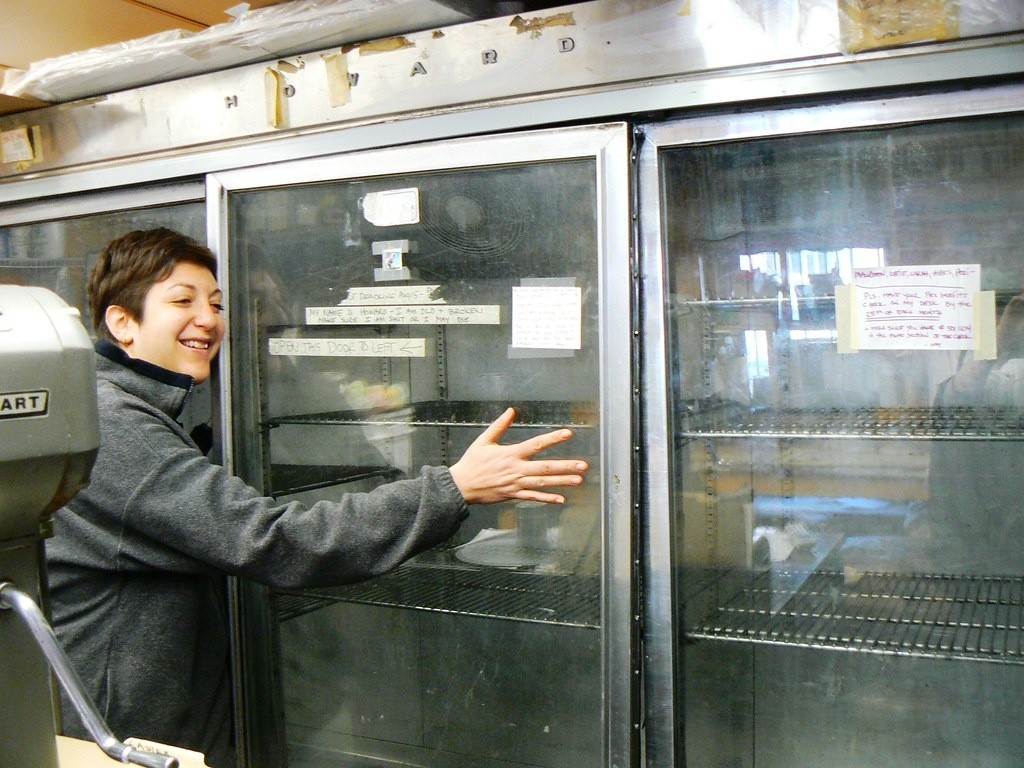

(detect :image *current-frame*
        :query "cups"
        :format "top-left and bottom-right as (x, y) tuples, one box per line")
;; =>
(515, 500), (549, 557)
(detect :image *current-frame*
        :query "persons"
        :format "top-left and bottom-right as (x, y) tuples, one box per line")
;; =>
(44, 226), (588, 768)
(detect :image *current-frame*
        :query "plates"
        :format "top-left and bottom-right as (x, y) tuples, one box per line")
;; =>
(454, 537), (544, 567)
